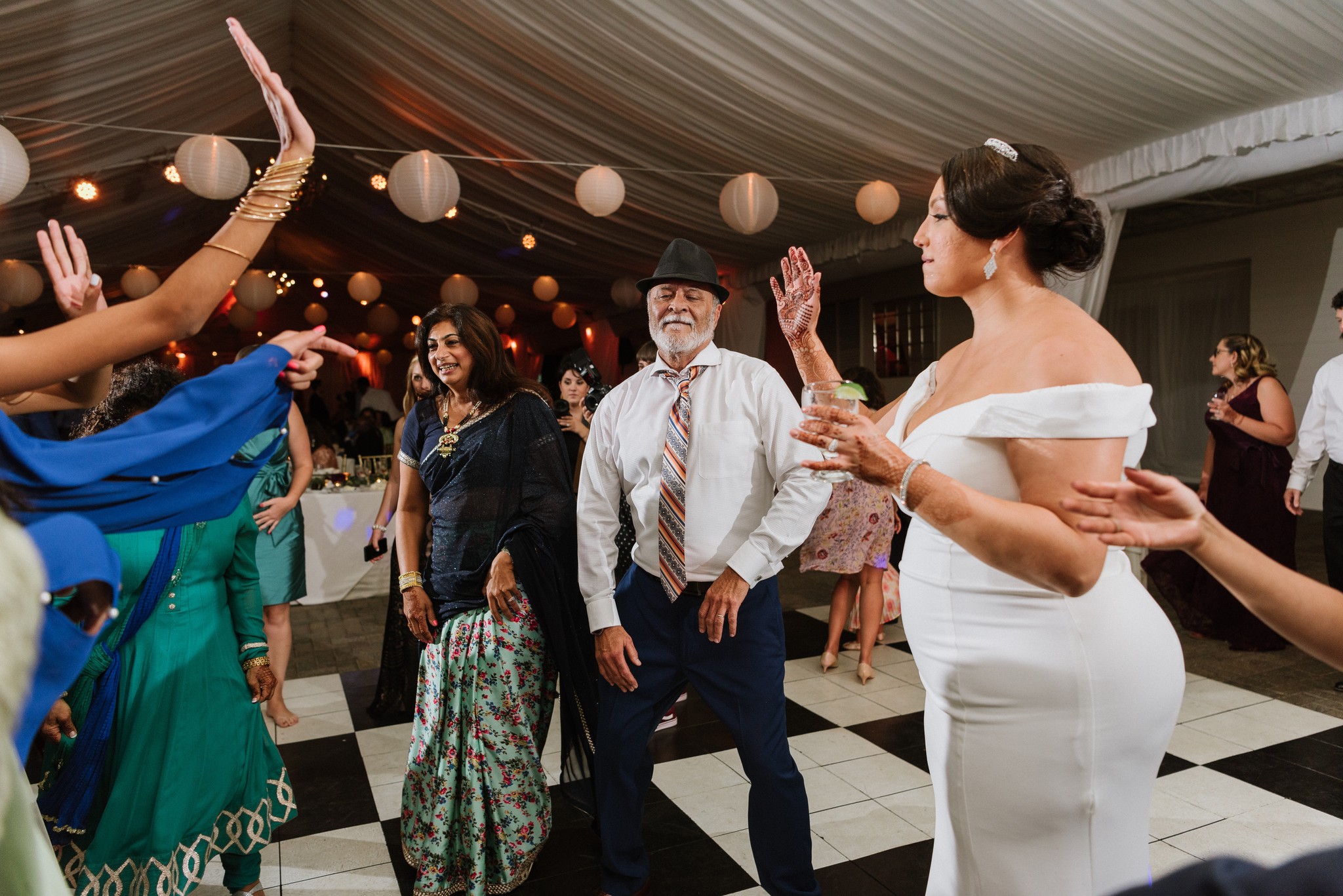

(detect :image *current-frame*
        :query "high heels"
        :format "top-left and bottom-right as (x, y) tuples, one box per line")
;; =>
(820, 650), (840, 675)
(857, 662), (877, 686)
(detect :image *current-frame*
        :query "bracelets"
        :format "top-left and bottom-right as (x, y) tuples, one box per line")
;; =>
(500, 546), (516, 565)
(371, 525), (387, 534)
(240, 641), (268, 652)
(899, 457), (931, 509)
(201, 241), (254, 266)
(228, 156), (315, 223)
(398, 570), (424, 594)
(242, 656), (271, 671)
(54, 691), (68, 700)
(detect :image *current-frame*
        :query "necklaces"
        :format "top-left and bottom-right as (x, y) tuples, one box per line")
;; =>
(1230, 385), (1239, 400)
(437, 389), (484, 459)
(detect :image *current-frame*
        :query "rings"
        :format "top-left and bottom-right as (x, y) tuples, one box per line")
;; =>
(716, 614), (726, 618)
(826, 438), (840, 452)
(566, 423), (570, 427)
(1216, 410), (1220, 414)
(785, 287), (793, 293)
(506, 595), (515, 602)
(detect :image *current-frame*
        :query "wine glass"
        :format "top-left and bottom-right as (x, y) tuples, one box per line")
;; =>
(802, 380), (859, 482)
(1210, 393), (1229, 420)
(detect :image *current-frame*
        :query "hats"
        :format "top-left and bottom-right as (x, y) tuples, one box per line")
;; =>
(635, 237), (732, 304)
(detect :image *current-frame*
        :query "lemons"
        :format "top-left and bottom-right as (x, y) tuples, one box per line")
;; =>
(833, 382), (868, 402)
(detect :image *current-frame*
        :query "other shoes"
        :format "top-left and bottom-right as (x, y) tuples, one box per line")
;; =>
(227, 878), (266, 896)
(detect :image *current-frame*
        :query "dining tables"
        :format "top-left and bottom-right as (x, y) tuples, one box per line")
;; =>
(288, 469), (396, 607)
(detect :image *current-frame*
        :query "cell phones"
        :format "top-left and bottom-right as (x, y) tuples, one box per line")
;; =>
(363, 537), (387, 562)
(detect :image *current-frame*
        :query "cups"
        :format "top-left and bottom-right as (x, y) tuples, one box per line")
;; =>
(380, 467), (388, 479)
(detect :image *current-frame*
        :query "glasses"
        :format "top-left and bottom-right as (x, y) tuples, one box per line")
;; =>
(1214, 347), (1236, 358)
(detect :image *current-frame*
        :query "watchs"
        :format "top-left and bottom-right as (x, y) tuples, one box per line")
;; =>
(593, 628), (604, 636)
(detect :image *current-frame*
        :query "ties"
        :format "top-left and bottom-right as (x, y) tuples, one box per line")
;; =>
(655, 364), (708, 603)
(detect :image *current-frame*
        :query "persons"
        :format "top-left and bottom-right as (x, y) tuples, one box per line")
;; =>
(1056, 467), (1343, 675)
(576, 237), (839, 896)
(393, 301), (581, 896)
(542, 357), (600, 480)
(1280, 285), (1343, 692)
(1196, 331), (1299, 652)
(840, 561), (904, 649)
(795, 365), (903, 686)
(30, 367), (305, 896)
(0, 17), (318, 417)
(366, 353), (439, 722)
(768, 135), (1188, 895)
(211, 341), (315, 727)
(578, 337), (693, 735)
(289, 358), (406, 481)
